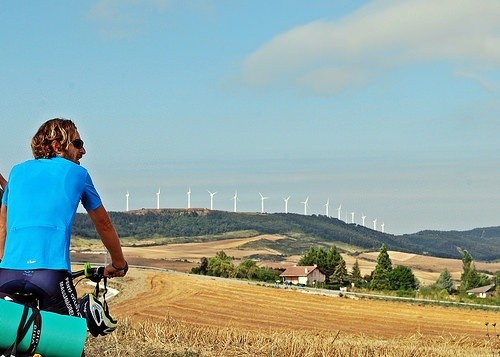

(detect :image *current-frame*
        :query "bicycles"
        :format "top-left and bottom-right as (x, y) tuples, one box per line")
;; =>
(0, 261), (126, 357)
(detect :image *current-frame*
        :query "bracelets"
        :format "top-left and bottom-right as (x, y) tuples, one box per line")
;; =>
(112, 262), (127, 270)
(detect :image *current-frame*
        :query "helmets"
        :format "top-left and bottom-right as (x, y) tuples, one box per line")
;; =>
(80, 293), (117, 337)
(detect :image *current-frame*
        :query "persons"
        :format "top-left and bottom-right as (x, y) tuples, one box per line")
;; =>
(0, 172), (8, 209)
(312, 280), (315, 287)
(0, 119), (128, 357)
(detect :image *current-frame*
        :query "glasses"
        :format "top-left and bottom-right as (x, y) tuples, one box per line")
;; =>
(71, 139), (83, 149)
